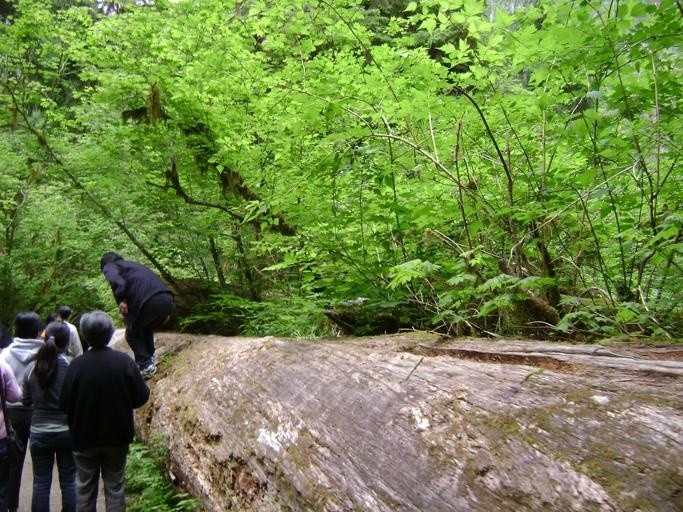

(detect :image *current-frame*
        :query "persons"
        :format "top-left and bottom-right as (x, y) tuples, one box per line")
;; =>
(0, 311), (45, 511)
(0, 355), (23, 512)
(53, 306), (82, 363)
(0, 316), (13, 349)
(22, 320), (76, 511)
(99, 250), (176, 383)
(37, 313), (56, 341)
(58, 309), (150, 512)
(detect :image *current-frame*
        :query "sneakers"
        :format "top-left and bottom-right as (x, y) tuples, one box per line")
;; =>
(139, 356), (157, 380)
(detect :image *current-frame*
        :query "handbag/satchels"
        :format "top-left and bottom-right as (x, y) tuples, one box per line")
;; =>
(9, 430), (26, 459)
